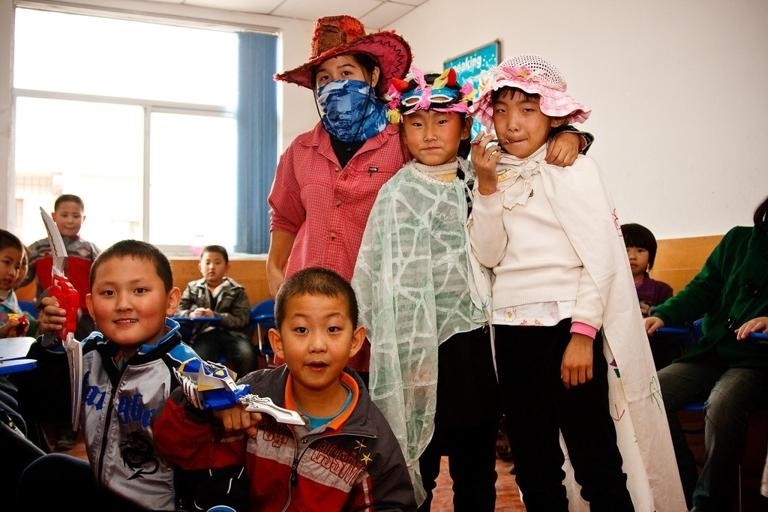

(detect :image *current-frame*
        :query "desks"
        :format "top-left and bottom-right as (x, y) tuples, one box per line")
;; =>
(170, 316), (222, 343)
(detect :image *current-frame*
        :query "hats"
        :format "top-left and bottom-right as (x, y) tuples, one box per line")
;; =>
(272, 15), (413, 91)
(471, 55), (592, 129)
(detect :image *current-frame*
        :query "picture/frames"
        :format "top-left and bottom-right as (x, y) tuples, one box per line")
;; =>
(441, 38), (503, 143)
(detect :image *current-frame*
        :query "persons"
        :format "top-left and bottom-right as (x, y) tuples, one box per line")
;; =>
(621, 224), (674, 318)
(0, 195), (102, 450)
(642, 197), (768, 512)
(153, 266), (417, 512)
(266, 50), (415, 390)
(173, 245), (258, 382)
(19, 240), (247, 512)
(466, 54), (636, 512)
(350, 73), (594, 512)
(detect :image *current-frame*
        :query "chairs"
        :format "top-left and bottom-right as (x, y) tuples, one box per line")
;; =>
(648, 315), (768, 434)
(250, 298), (276, 357)
(0, 336), (37, 374)
